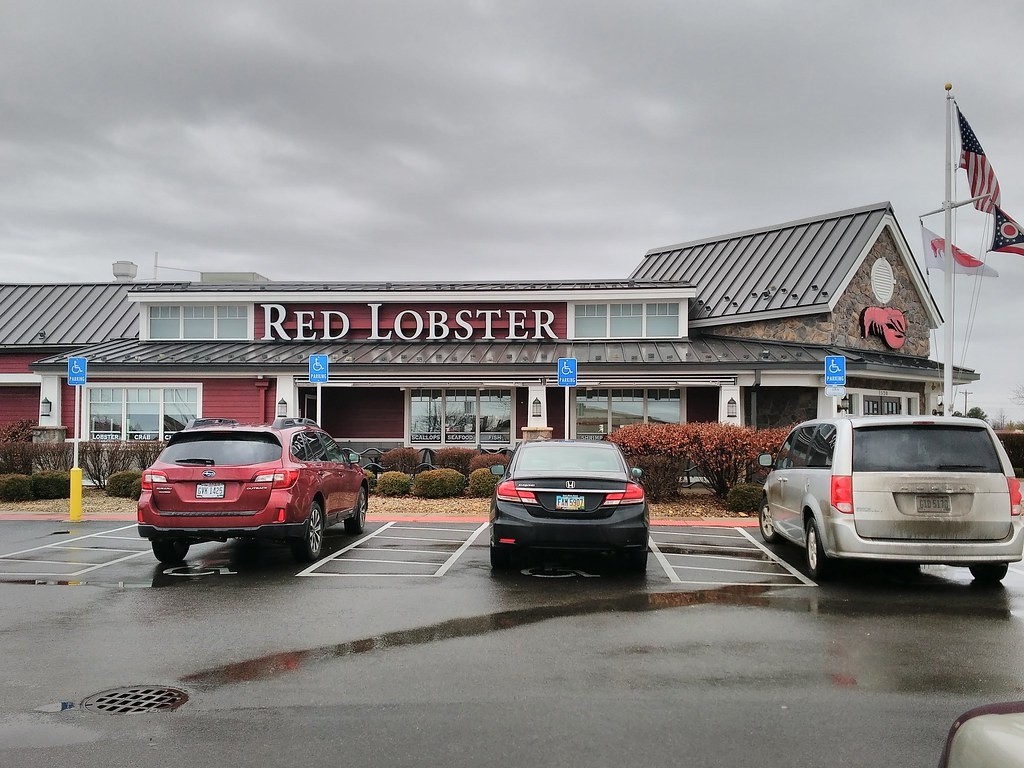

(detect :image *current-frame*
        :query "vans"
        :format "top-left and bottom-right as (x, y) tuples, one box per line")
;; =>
(759, 417), (1024, 582)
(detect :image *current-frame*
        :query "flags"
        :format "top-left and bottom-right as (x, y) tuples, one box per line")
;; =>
(953, 100), (1001, 215)
(921, 226), (998, 278)
(985, 204), (1023, 256)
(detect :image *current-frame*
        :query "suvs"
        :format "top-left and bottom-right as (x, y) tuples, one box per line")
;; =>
(137, 418), (368, 565)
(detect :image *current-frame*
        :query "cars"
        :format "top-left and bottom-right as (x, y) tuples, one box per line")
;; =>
(490, 438), (649, 581)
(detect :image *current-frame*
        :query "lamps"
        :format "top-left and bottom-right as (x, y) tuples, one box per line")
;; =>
(841, 395), (849, 411)
(726, 397), (737, 417)
(532, 397), (542, 417)
(937, 401), (944, 416)
(41, 397), (52, 416)
(278, 398), (287, 417)
